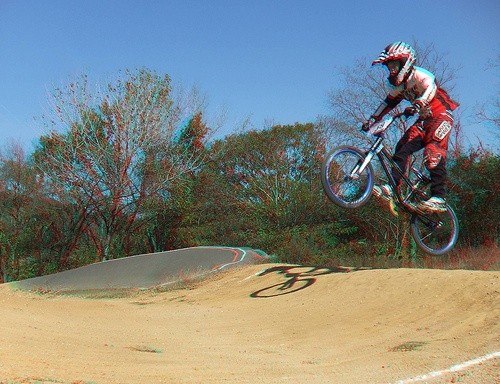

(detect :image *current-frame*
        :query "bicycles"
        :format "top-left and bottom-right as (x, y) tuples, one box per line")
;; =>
(320, 109), (460, 256)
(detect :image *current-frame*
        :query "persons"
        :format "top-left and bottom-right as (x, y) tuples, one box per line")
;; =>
(361, 41), (460, 212)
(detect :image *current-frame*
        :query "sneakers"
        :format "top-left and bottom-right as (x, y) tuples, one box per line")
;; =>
(418, 197), (447, 213)
(372, 183), (396, 202)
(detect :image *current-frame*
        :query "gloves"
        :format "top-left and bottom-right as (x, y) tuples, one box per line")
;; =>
(404, 103), (421, 118)
(362, 117), (376, 132)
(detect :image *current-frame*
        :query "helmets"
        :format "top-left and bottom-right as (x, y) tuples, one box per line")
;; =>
(372, 41), (417, 87)
